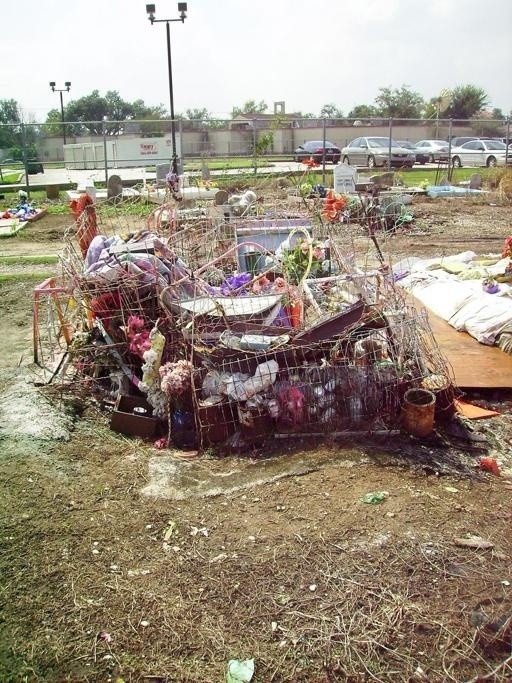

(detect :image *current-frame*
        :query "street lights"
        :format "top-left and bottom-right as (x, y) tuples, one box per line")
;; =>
(146, 0), (188, 189)
(49, 80), (73, 144)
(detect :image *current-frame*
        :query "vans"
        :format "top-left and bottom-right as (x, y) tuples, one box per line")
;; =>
(451, 136), (491, 147)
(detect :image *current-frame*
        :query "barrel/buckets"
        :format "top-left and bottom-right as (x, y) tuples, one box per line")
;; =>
(402, 387), (437, 439)
(199, 394), (230, 439)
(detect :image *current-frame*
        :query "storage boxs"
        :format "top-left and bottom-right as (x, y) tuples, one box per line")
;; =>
(110, 392), (162, 438)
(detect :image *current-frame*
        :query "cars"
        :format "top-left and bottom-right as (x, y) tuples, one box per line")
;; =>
(413, 139), (455, 162)
(490, 137), (512, 145)
(394, 140), (430, 164)
(292, 139), (342, 163)
(1, 158), (22, 164)
(508, 143), (512, 148)
(340, 135), (417, 168)
(449, 139), (512, 167)
